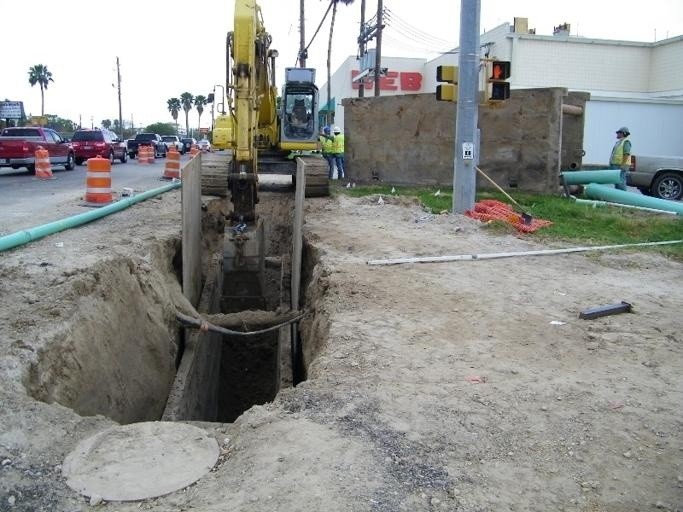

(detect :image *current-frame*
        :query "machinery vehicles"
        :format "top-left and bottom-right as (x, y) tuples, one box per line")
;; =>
(197, 0), (331, 301)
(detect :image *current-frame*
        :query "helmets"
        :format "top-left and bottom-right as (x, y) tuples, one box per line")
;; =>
(334, 127), (340, 132)
(324, 127), (329, 132)
(616, 127), (630, 134)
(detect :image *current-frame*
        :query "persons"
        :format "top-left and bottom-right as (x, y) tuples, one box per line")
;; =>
(319, 126), (346, 182)
(610, 126), (633, 190)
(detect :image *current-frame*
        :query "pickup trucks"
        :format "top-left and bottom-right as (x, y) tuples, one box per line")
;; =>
(127, 132), (169, 159)
(625, 155), (682, 200)
(0, 126), (76, 172)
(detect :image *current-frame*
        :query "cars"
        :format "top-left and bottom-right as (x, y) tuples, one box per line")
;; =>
(199, 141), (211, 152)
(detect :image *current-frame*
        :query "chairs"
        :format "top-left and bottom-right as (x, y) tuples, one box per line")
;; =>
(289, 98), (309, 132)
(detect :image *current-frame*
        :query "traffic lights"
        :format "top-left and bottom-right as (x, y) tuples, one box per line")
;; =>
(486, 59), (511, 104)
(435, 63), (459, 104)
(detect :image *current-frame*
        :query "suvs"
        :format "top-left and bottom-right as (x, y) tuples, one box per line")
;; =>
(162, 136), (185, 155)
(71, 129), (127, 164)
(182, 138), (200, 153)
